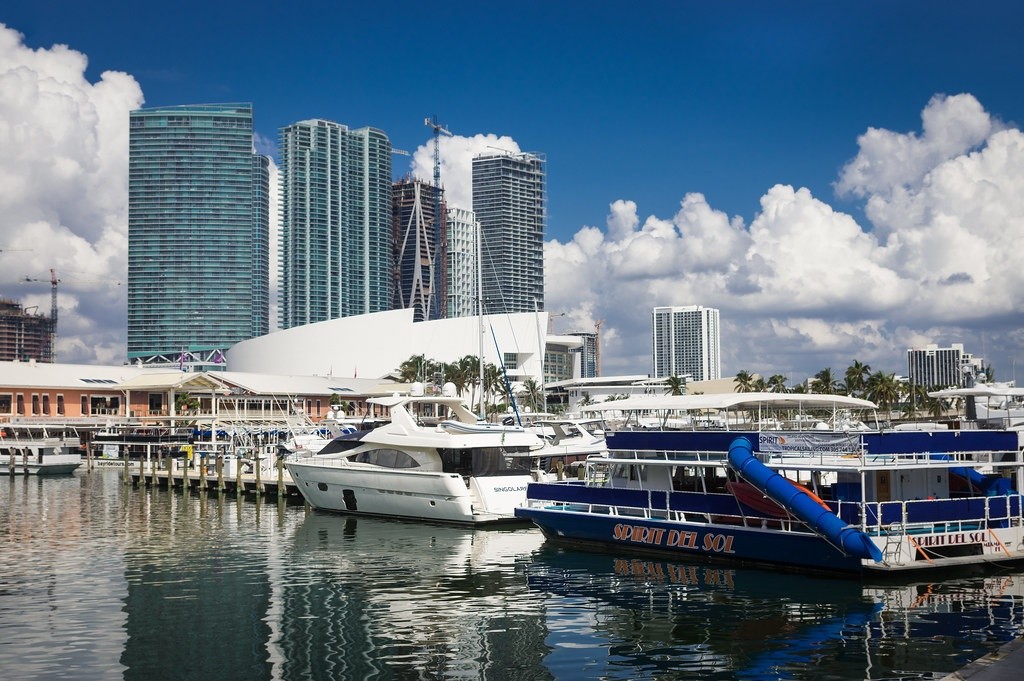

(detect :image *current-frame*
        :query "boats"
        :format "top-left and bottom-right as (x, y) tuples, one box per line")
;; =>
(1, 214), (1024, 587)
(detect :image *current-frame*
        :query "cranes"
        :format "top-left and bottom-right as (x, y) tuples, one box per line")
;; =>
(21, 267), (126, 363)
(423, 114), (454, 318)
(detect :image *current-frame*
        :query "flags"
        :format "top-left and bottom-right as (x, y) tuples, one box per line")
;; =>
(213, 353), (222, 364)
(179, 352), (185, 366)
